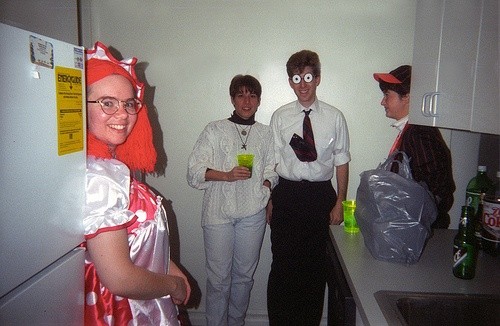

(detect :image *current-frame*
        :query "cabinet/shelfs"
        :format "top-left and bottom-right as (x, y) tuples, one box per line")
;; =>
(408, 0), (500, 135)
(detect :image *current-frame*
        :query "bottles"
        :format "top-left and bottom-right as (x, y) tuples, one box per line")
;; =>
(452, 206), (478, 280)
(465, 165), (495, 248)
(481, 171), (500, 257)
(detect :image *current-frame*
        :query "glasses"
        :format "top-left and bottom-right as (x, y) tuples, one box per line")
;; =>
(289, 73), (316, 84)
(86, 96), (142, 115)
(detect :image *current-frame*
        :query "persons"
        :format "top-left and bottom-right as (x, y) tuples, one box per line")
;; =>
(373, 65), (456, 228)
(186, 74), (280, 326)
(266, 49), (351, 326)
(79, 42), (191, 326)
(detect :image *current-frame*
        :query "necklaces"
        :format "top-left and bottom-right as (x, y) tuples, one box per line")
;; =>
(232, 114), (254, 151)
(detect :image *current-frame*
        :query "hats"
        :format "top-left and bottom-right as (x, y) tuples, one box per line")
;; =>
(374, 65), (412, 84)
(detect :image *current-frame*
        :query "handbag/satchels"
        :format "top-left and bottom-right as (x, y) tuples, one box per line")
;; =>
(354, 148), (437, 266)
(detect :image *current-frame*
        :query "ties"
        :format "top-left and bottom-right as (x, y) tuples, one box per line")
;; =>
(290, 109), (317, 161)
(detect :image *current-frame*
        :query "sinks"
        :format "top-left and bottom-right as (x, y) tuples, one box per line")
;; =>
(373, 289), (500, 326)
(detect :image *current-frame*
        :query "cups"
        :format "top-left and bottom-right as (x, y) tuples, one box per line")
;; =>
(236, 154), (255, 178)
(342, 200), (359, 233)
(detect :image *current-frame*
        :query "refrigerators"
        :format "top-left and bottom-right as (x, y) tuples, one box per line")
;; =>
(0, 22), (86, 326)
